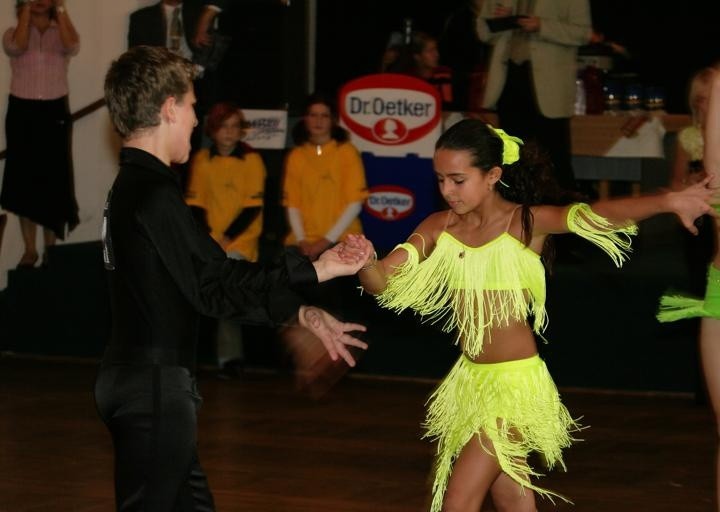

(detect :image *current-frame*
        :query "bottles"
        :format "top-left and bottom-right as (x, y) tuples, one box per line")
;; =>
(573, 81), (587, 116)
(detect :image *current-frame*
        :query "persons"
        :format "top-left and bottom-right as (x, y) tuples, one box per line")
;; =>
(127, 1), (720, 418)
(92, 46), (379, 512)
(0, 0), (80, 269)
(337, 114), (720, 510)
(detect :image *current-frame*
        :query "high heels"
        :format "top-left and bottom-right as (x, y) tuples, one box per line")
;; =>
(15, 250), (39, 273)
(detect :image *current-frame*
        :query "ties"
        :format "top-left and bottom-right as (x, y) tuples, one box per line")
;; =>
(167, 7), (184, 55)
(510, 1), (532, 67)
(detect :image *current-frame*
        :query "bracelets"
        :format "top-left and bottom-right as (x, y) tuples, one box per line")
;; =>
(55, 5), (66, 15)
